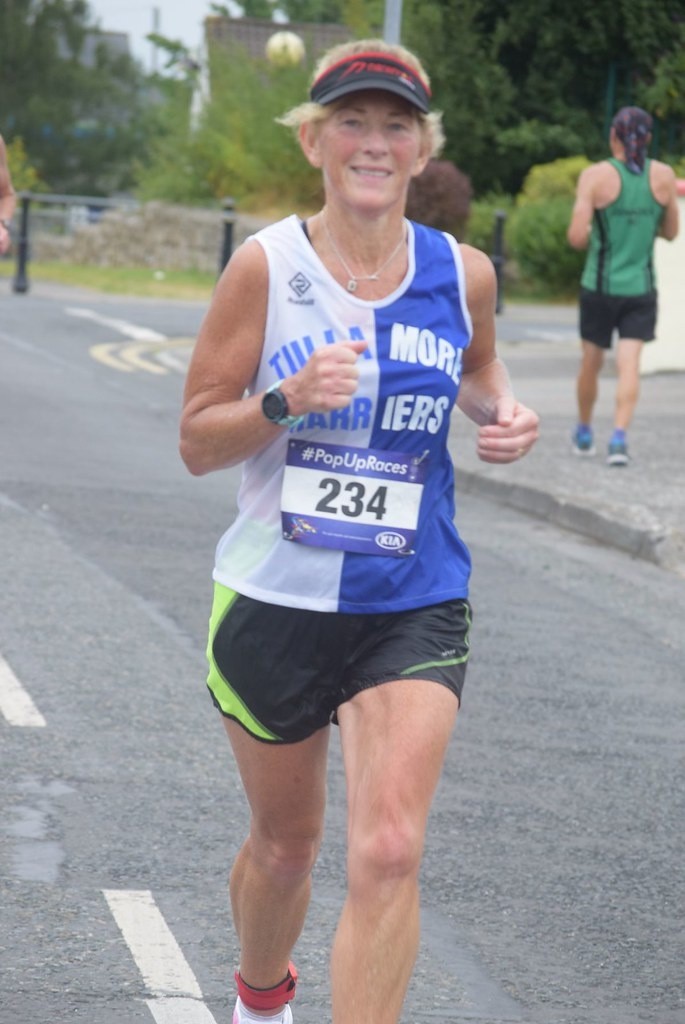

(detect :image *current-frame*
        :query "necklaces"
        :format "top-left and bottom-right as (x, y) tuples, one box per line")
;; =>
(321, 209), (409, 292)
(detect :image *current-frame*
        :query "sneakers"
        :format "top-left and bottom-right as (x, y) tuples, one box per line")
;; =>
(571, 426), (596, 456)
(607, 440), (629, 465)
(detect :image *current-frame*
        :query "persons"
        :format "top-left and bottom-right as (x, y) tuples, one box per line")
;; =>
(568, 106), (679, 466)
(0, 134), (16, 253)
(179, 40), (540, 1024)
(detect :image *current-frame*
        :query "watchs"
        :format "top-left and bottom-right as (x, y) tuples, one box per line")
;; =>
(262, 378), (304, 428)
(0, 219), (13, 231)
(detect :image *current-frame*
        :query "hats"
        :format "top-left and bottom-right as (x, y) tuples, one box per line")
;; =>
(310, 53), (431, 115)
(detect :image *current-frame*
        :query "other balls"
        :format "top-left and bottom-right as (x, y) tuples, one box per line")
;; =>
(265, 29), (307, 69)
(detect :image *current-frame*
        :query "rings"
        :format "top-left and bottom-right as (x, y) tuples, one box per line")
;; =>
(518, 448), (525, 458)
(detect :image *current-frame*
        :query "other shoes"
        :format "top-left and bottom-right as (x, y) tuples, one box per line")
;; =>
(232, 995), (293, 1024)
